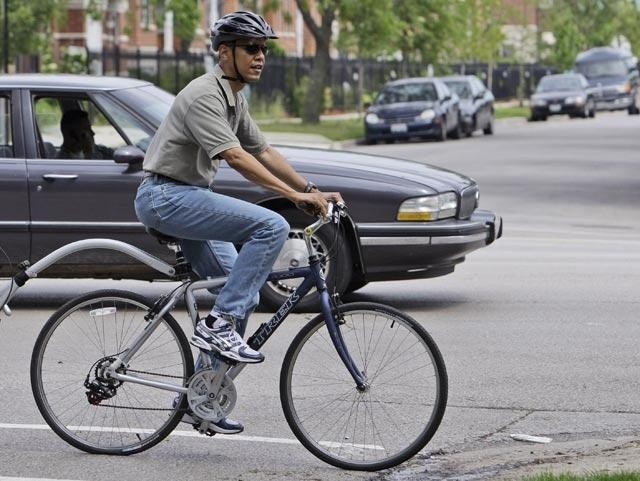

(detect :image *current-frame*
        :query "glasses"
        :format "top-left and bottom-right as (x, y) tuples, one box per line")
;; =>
(228, 44), (268, 56)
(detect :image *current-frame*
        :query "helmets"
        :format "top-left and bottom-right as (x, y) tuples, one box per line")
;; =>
(208, 9), (280, 52)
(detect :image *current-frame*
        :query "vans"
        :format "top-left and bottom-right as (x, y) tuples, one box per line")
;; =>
(573, 46), (640, 114)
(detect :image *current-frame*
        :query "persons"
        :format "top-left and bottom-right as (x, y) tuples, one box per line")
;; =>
(132, 6), (347, 438)
(56, 108), (106, 160)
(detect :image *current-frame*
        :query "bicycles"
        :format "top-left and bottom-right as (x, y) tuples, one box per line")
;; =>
(0, 201), (448, 472)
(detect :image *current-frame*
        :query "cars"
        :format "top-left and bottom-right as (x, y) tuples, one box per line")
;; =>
(529, 74), (596, 121)
(0, 73), (503, 313)
(437, 76), (496, 137)
(363, 77), (462, 145)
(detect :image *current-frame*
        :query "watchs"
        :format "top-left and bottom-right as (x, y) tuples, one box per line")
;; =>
(303, 181), (319, 195)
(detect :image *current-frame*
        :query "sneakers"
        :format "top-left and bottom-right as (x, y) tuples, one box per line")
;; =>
(190, 319), (266, 365)
(172, 395), (246, 435)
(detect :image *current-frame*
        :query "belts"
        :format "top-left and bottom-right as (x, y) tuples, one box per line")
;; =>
(141, 169), (173, 182)
